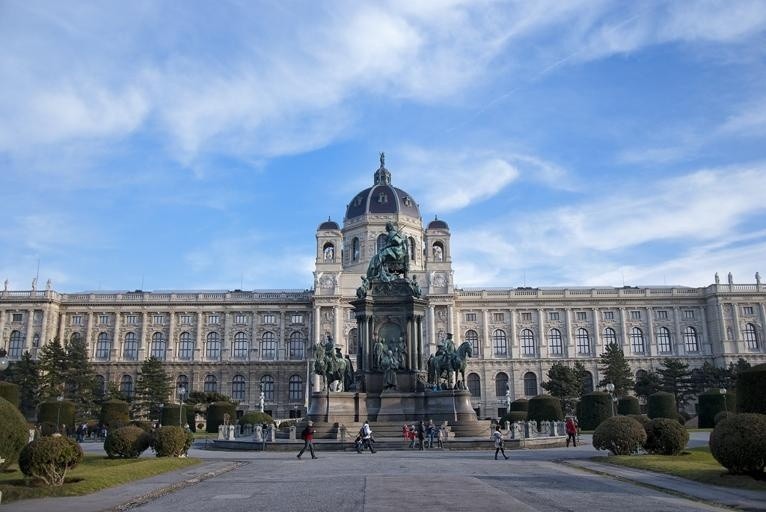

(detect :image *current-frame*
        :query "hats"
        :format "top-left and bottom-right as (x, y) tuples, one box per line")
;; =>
(307, 420), (315, 425)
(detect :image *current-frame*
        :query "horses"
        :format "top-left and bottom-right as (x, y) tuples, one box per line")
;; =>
(428, 342), (472, 390)
(310, 346), (350, 392)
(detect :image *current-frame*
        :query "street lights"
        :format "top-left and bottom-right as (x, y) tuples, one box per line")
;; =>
(719, 386), (728, 419)
(55, 394), (63, 434)
(0, 356), (10, 372)
(178, 386), (185, 428)
(606, 380), (614, 417)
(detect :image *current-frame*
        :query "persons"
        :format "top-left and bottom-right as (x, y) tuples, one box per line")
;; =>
(296, 420), (318, 459)
(358, 275), (370, 298)
(365, 222), (406, 283)
(493, 425), (509, 460)
(321, 335), (334, 357)
(566, 418), (578, 447)
(374, 336), (408, 391)
(411, 275), (422, 296)
(444, 332), (457, 355)
(335, 348), (342, 358)
(402, 419), (444, 451)
(75, 423), (83, 442)
(82, 422), (88, 440)
(357, 421), (376, 454)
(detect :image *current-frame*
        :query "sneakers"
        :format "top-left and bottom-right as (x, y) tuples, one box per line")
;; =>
(312, 456), (319, 459)
(296, 454), (303, 460)
(371, 451), (377, 454)
(505, 455), (510, 460)
(409, 445), (444, 451)
(566, 445), (577, 447)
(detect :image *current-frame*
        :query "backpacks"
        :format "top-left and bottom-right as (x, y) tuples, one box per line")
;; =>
(359, 425), (368, 437)
